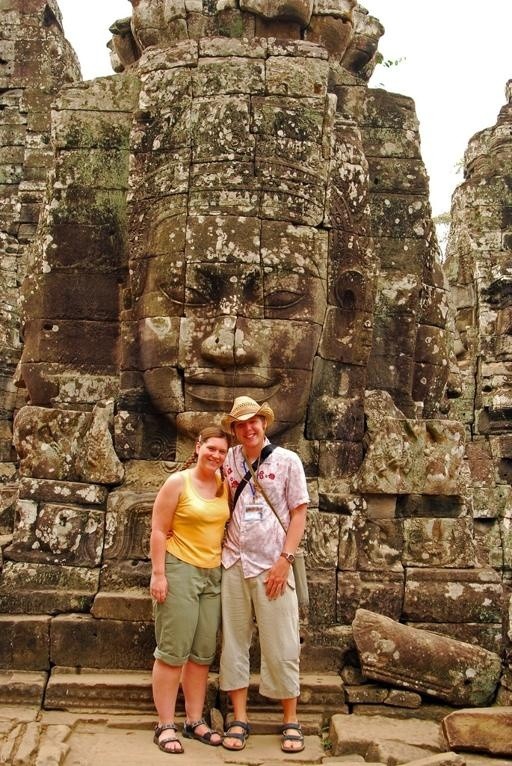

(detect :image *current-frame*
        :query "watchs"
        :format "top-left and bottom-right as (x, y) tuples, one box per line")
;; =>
(206, 396), (311, 753)
(280, 552), (296, 564)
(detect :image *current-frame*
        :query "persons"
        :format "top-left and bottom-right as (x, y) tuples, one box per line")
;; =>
(138, 213), (331, 445)
(150, 427), (231, 753)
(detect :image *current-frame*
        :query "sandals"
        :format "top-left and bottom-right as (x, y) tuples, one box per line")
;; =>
(222, 721), (250, 750)
(280, 723), (305, 753)
(153, 722), (184, 754)
(182, 719), (222, 750)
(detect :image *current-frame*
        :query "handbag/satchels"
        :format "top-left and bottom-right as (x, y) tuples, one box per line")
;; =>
(293, 551), (309, 601)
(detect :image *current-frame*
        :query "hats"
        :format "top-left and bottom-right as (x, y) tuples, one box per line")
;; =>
(220, 396), (274, 436)
